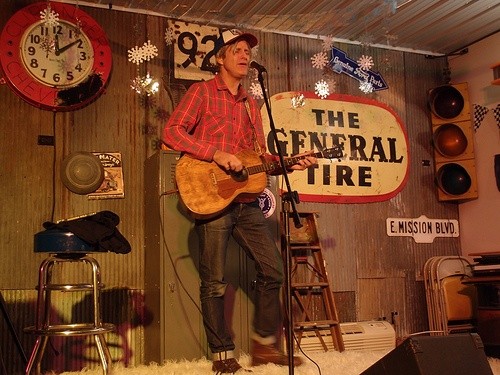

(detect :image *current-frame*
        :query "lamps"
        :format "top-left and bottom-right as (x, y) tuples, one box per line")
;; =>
(428, 82), (478, 201)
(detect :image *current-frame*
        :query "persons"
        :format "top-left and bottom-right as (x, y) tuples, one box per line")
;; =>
(162, 29), (319, 375)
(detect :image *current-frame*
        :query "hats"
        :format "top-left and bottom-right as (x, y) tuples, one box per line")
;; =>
(214, 28), (258, 55)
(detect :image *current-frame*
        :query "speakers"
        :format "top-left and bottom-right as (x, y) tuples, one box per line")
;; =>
(360, 332), (494, 375)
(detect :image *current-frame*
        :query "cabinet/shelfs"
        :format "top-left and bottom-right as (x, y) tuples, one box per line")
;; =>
(460, 276), (500, 352)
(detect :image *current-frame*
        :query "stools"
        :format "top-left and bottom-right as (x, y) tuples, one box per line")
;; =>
(22, 230), (117, 375)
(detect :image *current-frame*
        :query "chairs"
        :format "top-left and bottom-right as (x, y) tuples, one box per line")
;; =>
(422, 255), (479, 337)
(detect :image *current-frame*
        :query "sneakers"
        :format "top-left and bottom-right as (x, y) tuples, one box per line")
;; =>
(211, 359), (253, 375)
(252, 342), (302, 366)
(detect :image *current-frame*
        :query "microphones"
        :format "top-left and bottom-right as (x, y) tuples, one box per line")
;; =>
(249, 59), (266, 72)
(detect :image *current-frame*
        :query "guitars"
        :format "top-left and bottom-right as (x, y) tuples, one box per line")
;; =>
(175, 142), (344, 222)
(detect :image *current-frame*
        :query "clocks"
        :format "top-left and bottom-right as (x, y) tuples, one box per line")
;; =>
(0, 1), (113, 112)
